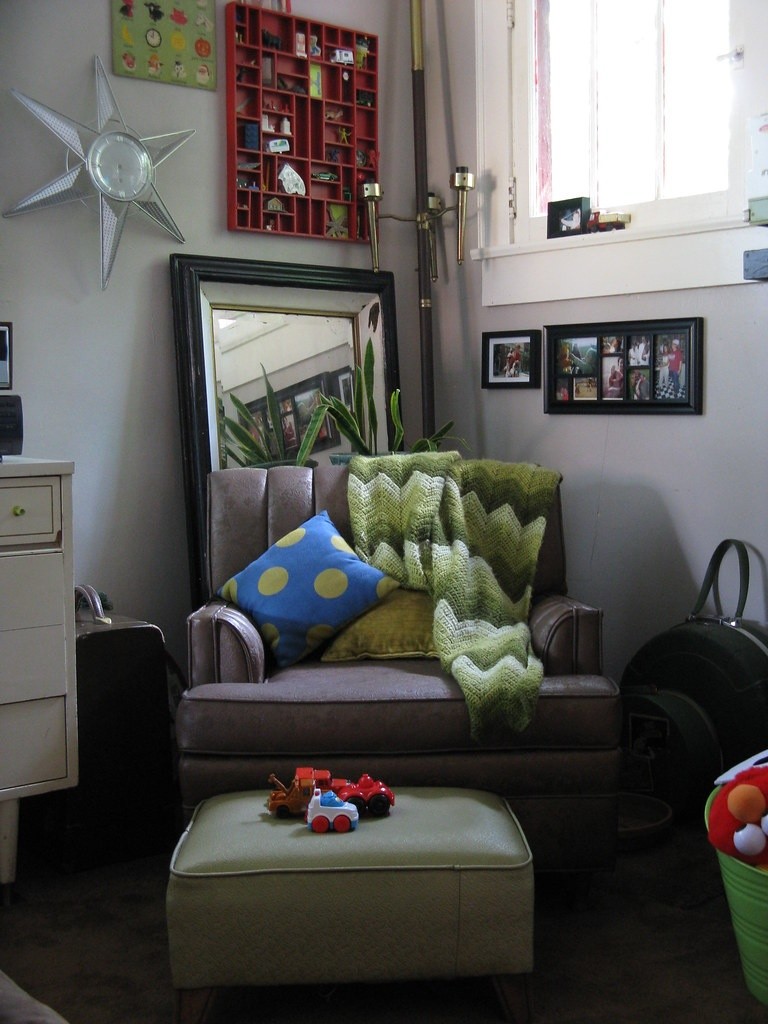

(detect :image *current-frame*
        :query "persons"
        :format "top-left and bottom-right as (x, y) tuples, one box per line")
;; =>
(338, 127), (351, 143)
(554, 336), (687, 401)
(503, 344), (522, 378)
(561, 208), (580, 230)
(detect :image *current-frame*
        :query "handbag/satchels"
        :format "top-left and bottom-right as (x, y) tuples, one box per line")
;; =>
(620, 539), (768, 821)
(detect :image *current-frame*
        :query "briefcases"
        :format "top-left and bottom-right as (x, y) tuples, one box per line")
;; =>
(20, 584), (173, 870)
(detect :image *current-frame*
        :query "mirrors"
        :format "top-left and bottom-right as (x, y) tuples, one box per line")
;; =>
(167, 252), (401, 596)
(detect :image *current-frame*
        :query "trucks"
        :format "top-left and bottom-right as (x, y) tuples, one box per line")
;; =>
(265, 774), (335, 819)
(294, 767), (354, 794)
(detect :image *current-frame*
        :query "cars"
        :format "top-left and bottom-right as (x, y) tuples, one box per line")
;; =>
(305, 788), (358, 835)
(335, 774), (396, 817)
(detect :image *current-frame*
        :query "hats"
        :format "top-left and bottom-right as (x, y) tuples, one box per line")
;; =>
(672, 339), (679, 345)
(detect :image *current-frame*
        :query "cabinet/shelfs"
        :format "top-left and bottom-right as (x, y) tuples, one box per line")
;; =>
(226, 1), (379, 244)
(0, 454), (80, 885)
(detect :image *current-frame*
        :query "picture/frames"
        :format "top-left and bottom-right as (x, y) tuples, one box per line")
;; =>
(544, 317), (703, 415)
(480, 330), (542, 391)
(234, 374), (341, 466)
(332, 366), (355, 414)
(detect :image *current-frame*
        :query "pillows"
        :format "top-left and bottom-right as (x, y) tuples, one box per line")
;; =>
(322, 588), (441, 663)
(217, 509), (398, 675)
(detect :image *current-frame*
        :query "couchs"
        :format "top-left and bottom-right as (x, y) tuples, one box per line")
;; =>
(172, 452), (623, 873)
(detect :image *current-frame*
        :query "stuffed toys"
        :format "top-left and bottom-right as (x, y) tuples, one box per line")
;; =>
(708, 765), (768, 868)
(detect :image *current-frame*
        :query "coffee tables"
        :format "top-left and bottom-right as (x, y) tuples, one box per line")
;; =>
(164, 787), (535, 994)
